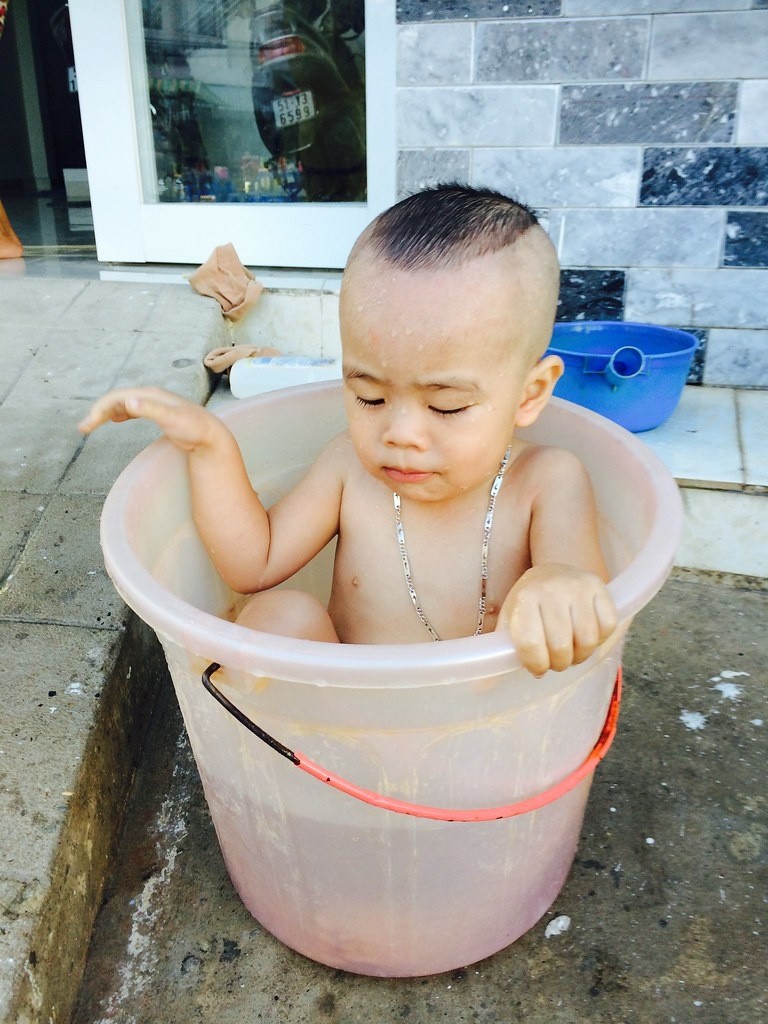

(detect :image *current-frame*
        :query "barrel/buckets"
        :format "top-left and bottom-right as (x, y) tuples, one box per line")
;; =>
(97, 377), (684, 979)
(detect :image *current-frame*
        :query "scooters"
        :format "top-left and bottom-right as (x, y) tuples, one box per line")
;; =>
(250, 3), (367, 201)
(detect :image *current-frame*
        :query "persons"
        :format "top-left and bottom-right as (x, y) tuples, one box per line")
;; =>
(74, 182), (619, 729)
(158, 49), (208, 174)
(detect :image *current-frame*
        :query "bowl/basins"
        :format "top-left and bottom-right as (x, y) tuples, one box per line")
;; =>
(541, 320), (700, 433)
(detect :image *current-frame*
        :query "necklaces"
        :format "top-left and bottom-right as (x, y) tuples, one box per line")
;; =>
(393, 445), (510, 642)
